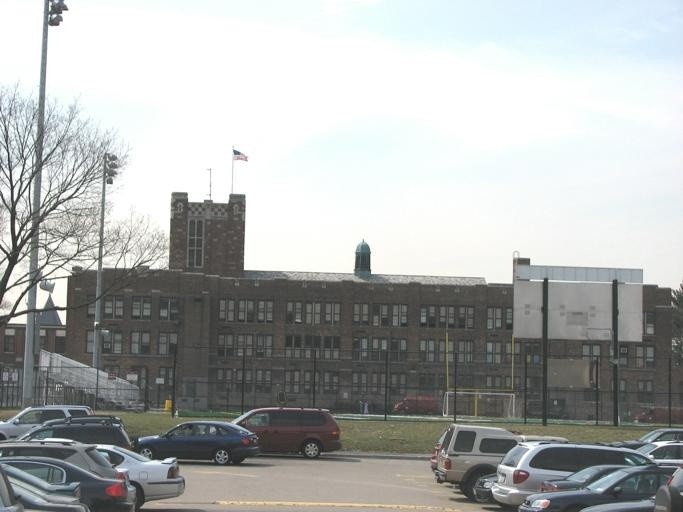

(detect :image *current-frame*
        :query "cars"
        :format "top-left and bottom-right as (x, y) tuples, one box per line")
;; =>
(427, 424), (683, 511)
(0, 400), (187, 512)
(125, 419), (261, 466)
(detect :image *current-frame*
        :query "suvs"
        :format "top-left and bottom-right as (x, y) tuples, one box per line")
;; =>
(224, 406), (344, 461)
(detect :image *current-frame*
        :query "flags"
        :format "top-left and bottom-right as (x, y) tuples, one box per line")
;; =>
(233, 149), (248, 162)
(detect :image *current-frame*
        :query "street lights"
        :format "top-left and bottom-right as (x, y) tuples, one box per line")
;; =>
(93, 148), (118, 371)
(20, 0), (70, 412)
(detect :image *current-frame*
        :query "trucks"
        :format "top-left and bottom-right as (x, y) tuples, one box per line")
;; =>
(393, 395), (439, 415)
(632, 406), (683, 425)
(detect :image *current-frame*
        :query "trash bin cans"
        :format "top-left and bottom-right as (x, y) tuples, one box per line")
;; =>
(163, 400), (172, 411)
